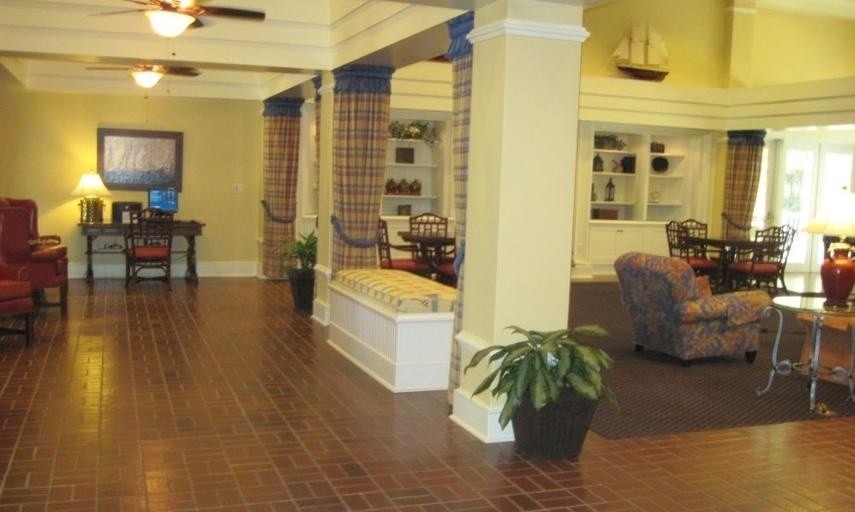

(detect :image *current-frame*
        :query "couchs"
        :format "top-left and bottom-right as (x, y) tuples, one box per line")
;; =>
(614, 252), (772, 367)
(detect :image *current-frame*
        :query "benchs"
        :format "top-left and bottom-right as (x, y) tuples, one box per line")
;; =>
(327, 270), (458, 393)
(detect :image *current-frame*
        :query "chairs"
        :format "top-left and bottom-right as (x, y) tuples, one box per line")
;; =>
(0, 208), (35, 347)
(378, 211), (457, 287)
(122, 208), (174, 291)
(1, 197), (68, 315)
(665, 220), (797, 295)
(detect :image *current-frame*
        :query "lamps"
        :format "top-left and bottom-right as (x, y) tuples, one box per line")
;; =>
(804, 189), (855, 309)
(130, 65), (167, 88)
(143, 2), (204, 36)
(70, 173), (112, 225)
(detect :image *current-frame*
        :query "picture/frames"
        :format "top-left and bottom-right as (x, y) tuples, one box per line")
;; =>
(97, 128), (183, 192)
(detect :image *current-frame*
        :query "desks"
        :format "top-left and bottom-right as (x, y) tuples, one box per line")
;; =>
(755, 296), (855, 414)
(76, 220), (206, 286)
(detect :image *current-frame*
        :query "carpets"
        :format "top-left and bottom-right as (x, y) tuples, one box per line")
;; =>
(570, 280), (854, 440)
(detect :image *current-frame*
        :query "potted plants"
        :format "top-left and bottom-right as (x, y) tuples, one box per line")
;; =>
(463, 324), (620, 462)
(275, 229), (317, 309)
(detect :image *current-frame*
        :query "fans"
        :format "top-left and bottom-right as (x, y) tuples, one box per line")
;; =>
(85, 64), (201, 77)
(83, 0), (266, 31)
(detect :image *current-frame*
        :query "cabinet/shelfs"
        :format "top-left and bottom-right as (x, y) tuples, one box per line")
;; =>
(646, 223), (702, 257)
(589, 222), (642, 280)
(590, 150), (684, 207)
(799, 311), (855, 387)
(379, 137), (437, 198)
